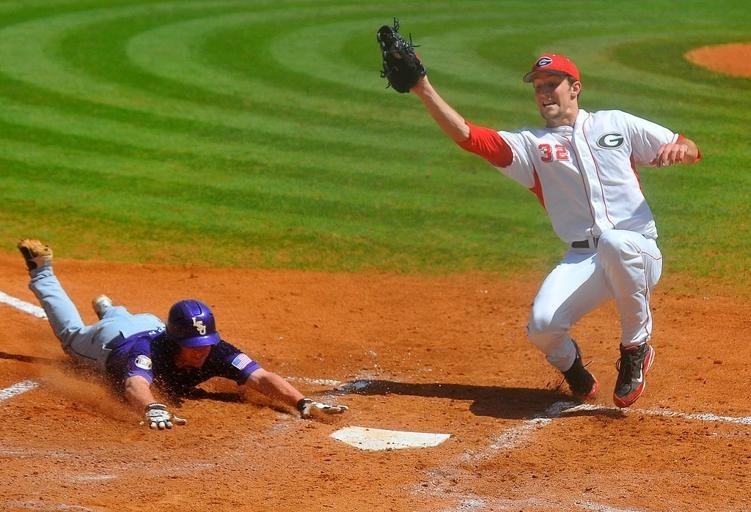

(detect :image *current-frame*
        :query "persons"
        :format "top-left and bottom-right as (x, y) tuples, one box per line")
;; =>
(15, 237), (349, 430)
(377, 18), (702, 408)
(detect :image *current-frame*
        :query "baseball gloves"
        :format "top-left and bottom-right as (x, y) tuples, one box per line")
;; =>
(376, 23), (426, 93)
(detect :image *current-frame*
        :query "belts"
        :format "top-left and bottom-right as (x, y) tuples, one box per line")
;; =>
(571, 239), (599, 249)
(105, 334), (125, 350)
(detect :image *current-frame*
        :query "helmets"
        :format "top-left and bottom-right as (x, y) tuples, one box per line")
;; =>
(166, 299), (220, 348)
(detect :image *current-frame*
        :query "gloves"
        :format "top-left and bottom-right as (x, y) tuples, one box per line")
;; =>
(298, 397), (347, 418)
(141, 402), (186, 430)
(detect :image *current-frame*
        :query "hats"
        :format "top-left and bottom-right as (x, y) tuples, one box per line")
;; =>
(522, 55), (580, 81)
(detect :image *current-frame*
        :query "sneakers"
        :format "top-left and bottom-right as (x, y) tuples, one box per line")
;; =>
(93, 294), (113, 319)
(560, 338), (598, 398)
(18, 239), (54, 270)
(613, 341), (654, 406)
